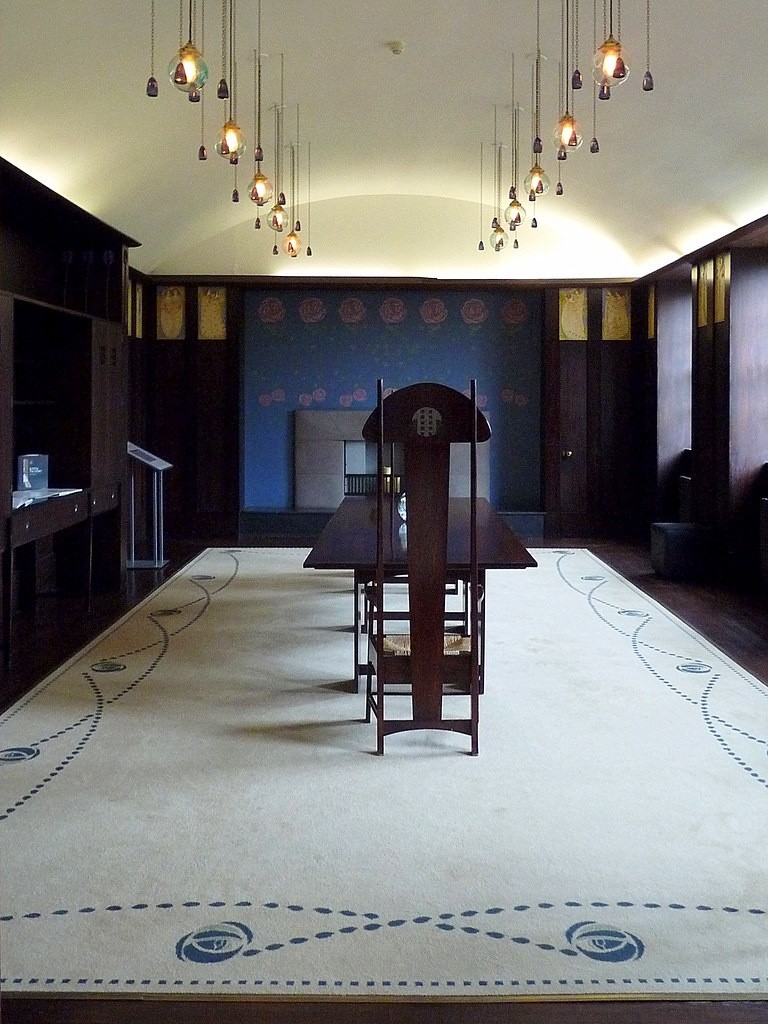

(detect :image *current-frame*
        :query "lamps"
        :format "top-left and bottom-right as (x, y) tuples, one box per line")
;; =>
(145, 0), (313, 259)
(478, 0), (655, 253)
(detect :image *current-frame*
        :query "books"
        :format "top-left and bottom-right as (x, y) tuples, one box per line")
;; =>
(18, 454), (48, 491)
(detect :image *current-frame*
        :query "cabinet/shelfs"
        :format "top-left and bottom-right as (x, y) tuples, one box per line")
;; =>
(0, 290), (129, 640)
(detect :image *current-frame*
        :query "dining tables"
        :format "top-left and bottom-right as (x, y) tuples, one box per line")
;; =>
(302, 497), (539, 693)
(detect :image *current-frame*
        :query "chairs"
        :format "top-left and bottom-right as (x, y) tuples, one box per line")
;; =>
(362, 377), (493, 756)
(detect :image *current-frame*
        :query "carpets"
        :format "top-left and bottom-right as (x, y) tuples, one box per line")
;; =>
(0, 547), (768, 1002)
